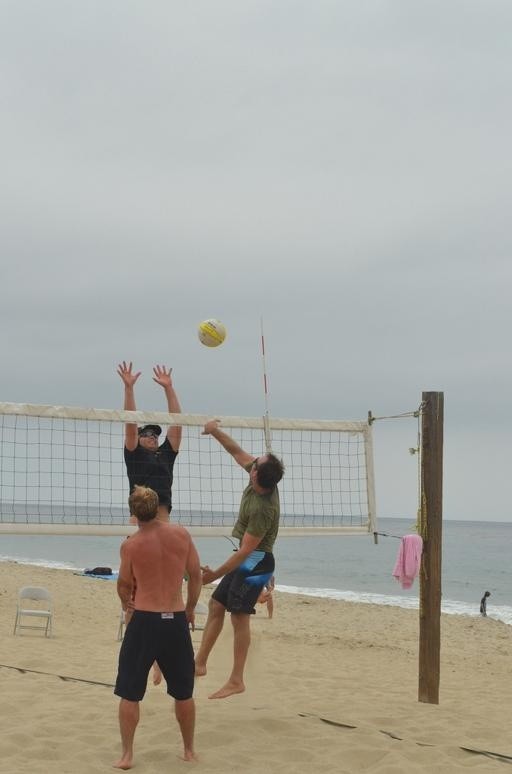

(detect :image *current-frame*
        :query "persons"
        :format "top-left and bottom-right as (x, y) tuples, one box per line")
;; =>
(115, 360), (184, 686)
(193, 418), (285, 700)
(227, 575), (275, 618)
(479, 590), (491, 616)
(115, 482), (201, 771)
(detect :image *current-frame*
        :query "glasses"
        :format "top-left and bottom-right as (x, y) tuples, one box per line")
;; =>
(139, 431), (158, 439)
(252, 457), (259, 472)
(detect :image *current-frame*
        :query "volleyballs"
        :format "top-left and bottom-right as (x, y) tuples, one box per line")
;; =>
(198, 319), (226, 347)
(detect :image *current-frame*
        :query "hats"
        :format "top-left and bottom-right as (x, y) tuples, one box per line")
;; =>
(137, 424), (162, 434)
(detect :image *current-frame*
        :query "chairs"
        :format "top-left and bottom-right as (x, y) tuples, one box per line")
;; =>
(118, 610), (126, 640)
(188, 603), (209, 642)
(14, 586), (53, 638)
